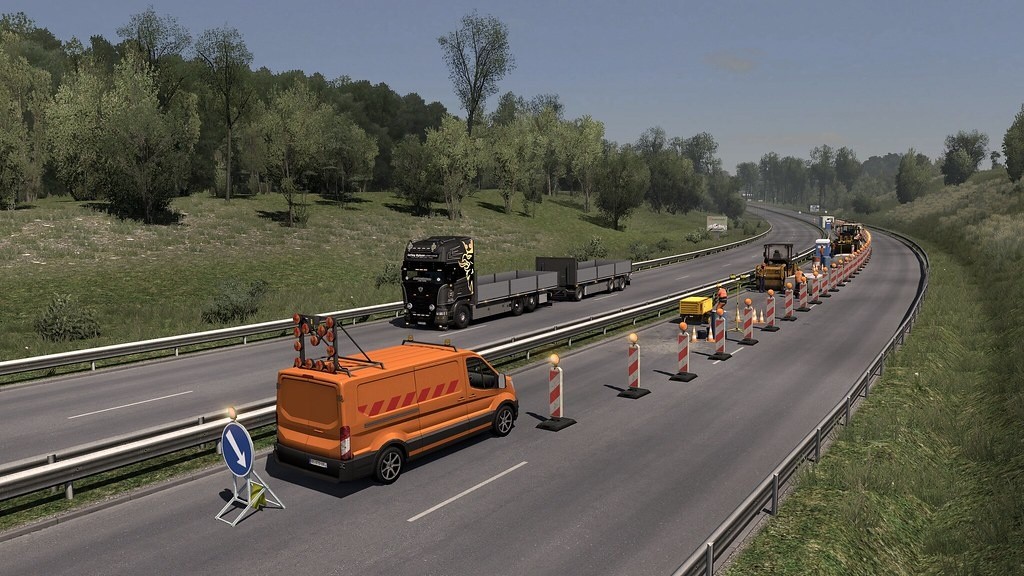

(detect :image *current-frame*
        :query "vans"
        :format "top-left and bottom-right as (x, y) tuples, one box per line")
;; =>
(270, 313), (519, 486)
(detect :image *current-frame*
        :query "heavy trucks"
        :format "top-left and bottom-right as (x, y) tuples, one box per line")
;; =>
(395, 235), (633, 332)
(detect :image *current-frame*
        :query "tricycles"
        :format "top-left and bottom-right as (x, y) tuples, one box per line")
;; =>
(678, 291), (723, 324)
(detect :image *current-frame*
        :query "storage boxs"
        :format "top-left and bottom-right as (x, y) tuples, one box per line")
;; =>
(825, 220), (831, 229)
(816, 239), (830, 256)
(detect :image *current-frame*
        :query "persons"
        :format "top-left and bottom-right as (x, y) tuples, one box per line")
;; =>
(757, 262), (766, 294)
(794, 266), (804, 298)
(819, 245), (826, 269)
(830, 241), (835, 259)
(714, 283), (727, 310)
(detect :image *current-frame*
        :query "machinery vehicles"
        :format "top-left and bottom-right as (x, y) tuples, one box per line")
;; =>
(830, 223), (864, 255)
(755, 243), (800, 294)
